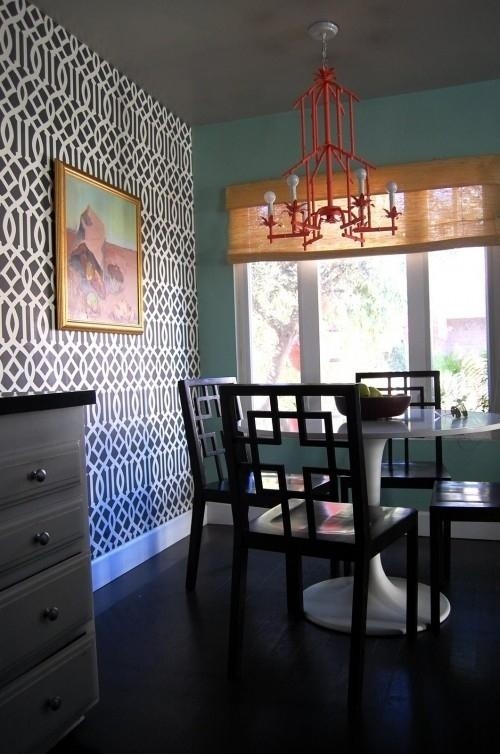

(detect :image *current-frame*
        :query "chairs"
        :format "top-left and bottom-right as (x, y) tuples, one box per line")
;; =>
(337, 370), (454, 600)
(218, 383), (419, 697)
(178, 376), (339, 595)
(430, 480), (499, 641)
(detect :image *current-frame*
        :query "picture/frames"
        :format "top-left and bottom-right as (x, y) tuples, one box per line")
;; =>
(54, 158), (145, 335)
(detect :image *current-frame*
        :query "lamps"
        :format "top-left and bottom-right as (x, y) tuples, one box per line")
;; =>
(260, 20), (403, 252)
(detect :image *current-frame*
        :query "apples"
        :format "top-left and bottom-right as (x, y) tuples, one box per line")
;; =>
(356, 383), (370, 398)
(369, 387), (382, 397)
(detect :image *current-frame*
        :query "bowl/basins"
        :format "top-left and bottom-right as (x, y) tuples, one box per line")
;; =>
(334, 394), (410, 418)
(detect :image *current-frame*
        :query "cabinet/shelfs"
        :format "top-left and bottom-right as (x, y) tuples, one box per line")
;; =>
(1, 390), (100, 753)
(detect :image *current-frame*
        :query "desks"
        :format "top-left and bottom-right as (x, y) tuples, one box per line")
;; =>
(236, 410), (500, 636)
